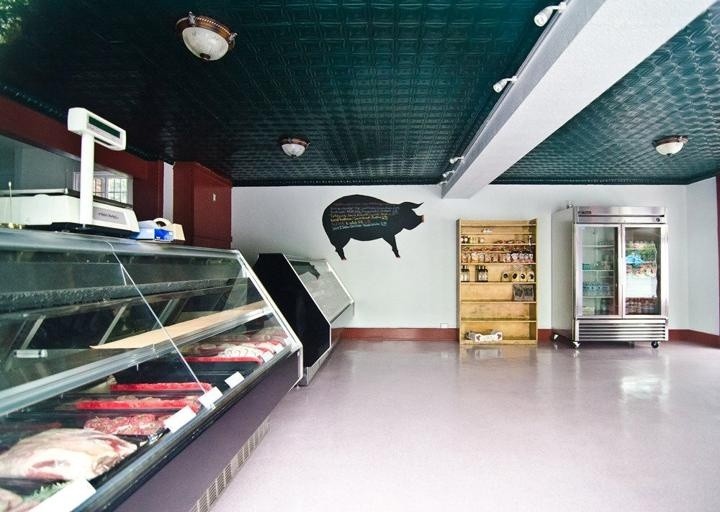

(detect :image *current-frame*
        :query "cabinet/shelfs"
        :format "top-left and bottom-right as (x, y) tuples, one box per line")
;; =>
(245, 254), (355, 388)
(456, 217), (539, 345)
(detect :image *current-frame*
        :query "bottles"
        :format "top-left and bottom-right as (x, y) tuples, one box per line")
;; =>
(477, 265), (489, 282)
(600, 298), (614, 315)
(626, 298), (660, 315)
(626, 239), (656, 260)
(461, 265), (470, 282)
(583, 281), (614, 296)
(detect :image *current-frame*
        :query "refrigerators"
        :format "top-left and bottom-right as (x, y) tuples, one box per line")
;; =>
(570, 205), (669, 349)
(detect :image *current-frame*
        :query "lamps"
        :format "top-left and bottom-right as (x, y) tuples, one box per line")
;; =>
(534, 1), (567, 27)
(493, 75), (517, 93)
(440, 155), (465, 185)
(281, 137), (311, 160)
(652, 135), (689, 158)
(176, 11), (239, 62)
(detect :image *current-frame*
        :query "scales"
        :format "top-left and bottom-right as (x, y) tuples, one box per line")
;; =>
(0, 106), (141, 238)
